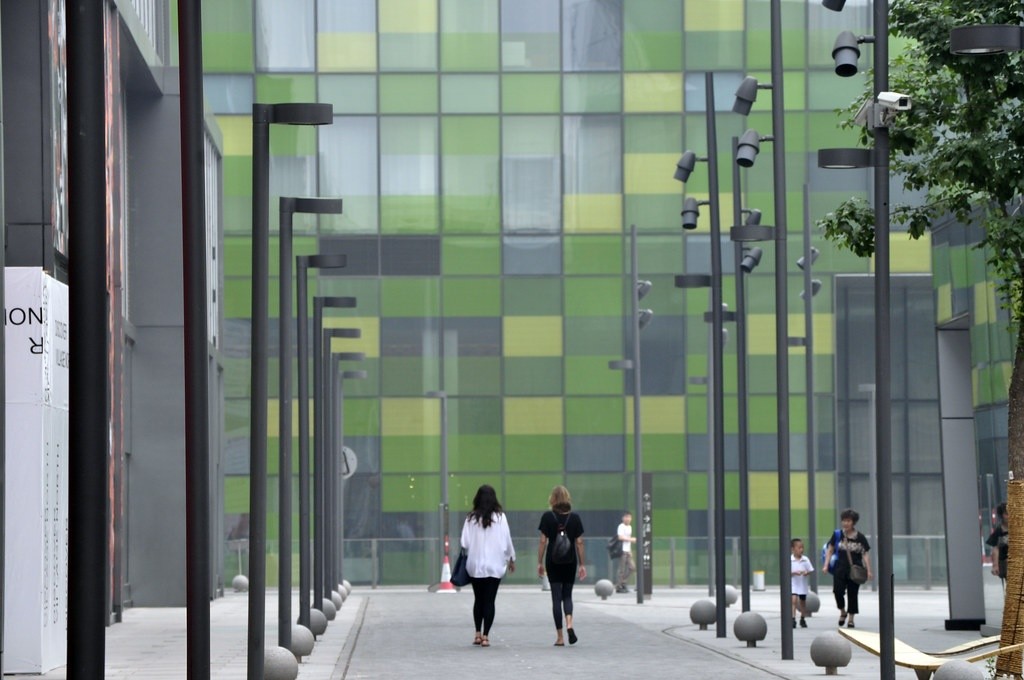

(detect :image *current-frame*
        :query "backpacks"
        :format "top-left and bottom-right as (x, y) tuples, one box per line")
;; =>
(551, 507), (575, 563)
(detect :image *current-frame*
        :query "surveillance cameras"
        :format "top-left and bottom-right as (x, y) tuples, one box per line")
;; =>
(877, 91), (912, 110)
(854, 98), (874, 129)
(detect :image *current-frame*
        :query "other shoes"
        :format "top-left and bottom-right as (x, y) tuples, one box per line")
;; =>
(800, 619), (807, 627)
(614, 584), (627, 593)
(838, 612), (847, 626)
(848, 621), (854, 628)
(793, 618), (796, 628)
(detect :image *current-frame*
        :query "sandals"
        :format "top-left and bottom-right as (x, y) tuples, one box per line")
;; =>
(481, 638), (489, 647)
(473, 637), (481, 644)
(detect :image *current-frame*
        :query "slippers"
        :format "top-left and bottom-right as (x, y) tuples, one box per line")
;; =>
(554, 641), (565, 646)
(567, 626), (577, 644)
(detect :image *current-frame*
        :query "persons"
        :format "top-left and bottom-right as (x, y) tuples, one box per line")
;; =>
(460, 484), (516, 647)
(985, 503), (1009, 596)
(615, 511), (636, 593)
(791, 538), (815, 628)
(822, 509), (873, 628)
(537, 484), (587, 645)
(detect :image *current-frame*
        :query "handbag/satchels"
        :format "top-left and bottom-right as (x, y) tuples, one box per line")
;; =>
(823, 529), (840, 575)
(607, 534), (622, 559)
(850, 565), (867, 584)
(450, 544), (473, 587)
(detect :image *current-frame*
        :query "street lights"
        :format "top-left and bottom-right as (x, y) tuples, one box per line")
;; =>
(700, 134), (766, 615)
(819, 1), (918, 680)
(724, 0), (796, 662)
(422, 390), (450, 578)
(322, 325), (363, 602)
(275, 191), (346, 653)
(785, 183), (825, 592)
(674, 70), (732, 640)
(295, 251), (350, 629)
(243, 97), (335, 680)
(330, 349), (367, 593)
(606, 222), (647, 603)
(337, 369), (368, 584)
(311, 296), (357, 612)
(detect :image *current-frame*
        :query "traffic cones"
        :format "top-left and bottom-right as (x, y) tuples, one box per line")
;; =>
(432, 554), (458, 595)
